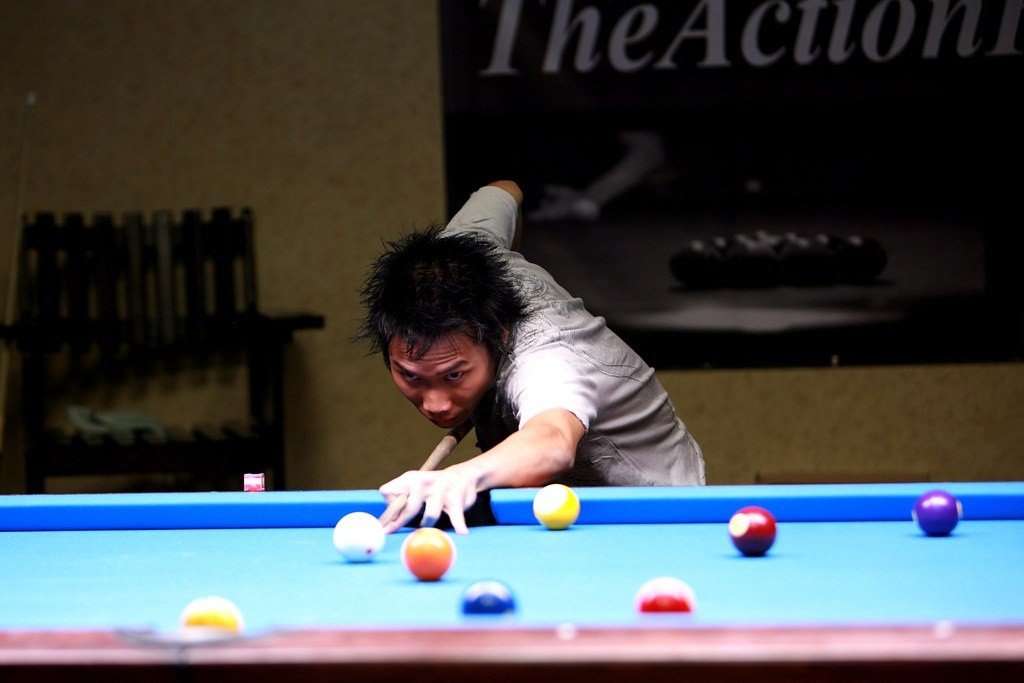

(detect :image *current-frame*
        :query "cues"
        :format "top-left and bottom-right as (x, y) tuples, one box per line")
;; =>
(378, 418), (474, 528)
(0, 87), (37, 456)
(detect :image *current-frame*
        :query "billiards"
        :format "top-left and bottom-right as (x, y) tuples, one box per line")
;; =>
(461, 579), (514, 617)
(728, 506), (777, 557)
(401, 527), (455, 580)
(181, 595), (245, 640)
(912, 491), (963, 536)
(637, 578), (694, 615)
(332, 511), (385, 563)
(664, 221), (889, 288)
(532, 482), (581, 530)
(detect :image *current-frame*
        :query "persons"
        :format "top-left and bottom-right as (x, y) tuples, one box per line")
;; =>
(528, 125), (664, 224)
(366, 180), (705, 534)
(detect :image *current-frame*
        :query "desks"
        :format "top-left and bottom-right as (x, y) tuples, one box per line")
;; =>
(0, 482), (1024, 683)
